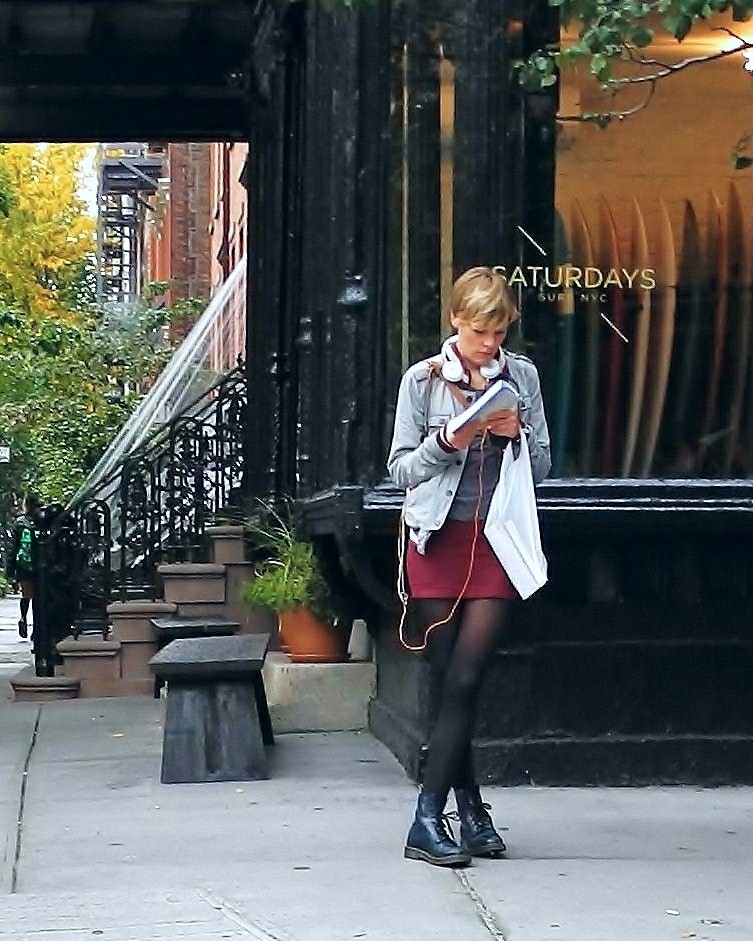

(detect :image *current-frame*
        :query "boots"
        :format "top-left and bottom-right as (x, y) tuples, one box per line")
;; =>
(405, 794), (471, 865)
(454, 783), (505, 857)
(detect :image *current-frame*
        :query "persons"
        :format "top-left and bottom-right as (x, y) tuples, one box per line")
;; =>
(6, 495), (42, 638)
(385, 267), (552, 866)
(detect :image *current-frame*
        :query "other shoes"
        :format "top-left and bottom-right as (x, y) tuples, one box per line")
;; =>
(18, 619), (27, 638)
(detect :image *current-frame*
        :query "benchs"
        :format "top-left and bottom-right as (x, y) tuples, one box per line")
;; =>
(145, 614), (275, 786)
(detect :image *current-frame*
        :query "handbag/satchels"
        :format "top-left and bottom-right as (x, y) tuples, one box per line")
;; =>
(482, 427), (548, 600)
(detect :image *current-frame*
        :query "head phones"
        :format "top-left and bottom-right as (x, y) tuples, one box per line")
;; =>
(440, 334), (506, 383)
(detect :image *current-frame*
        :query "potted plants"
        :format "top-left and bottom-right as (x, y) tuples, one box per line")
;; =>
(225, 492), (350, 664)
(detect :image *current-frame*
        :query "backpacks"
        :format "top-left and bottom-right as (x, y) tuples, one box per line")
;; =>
(15, 524), (32, 573)
